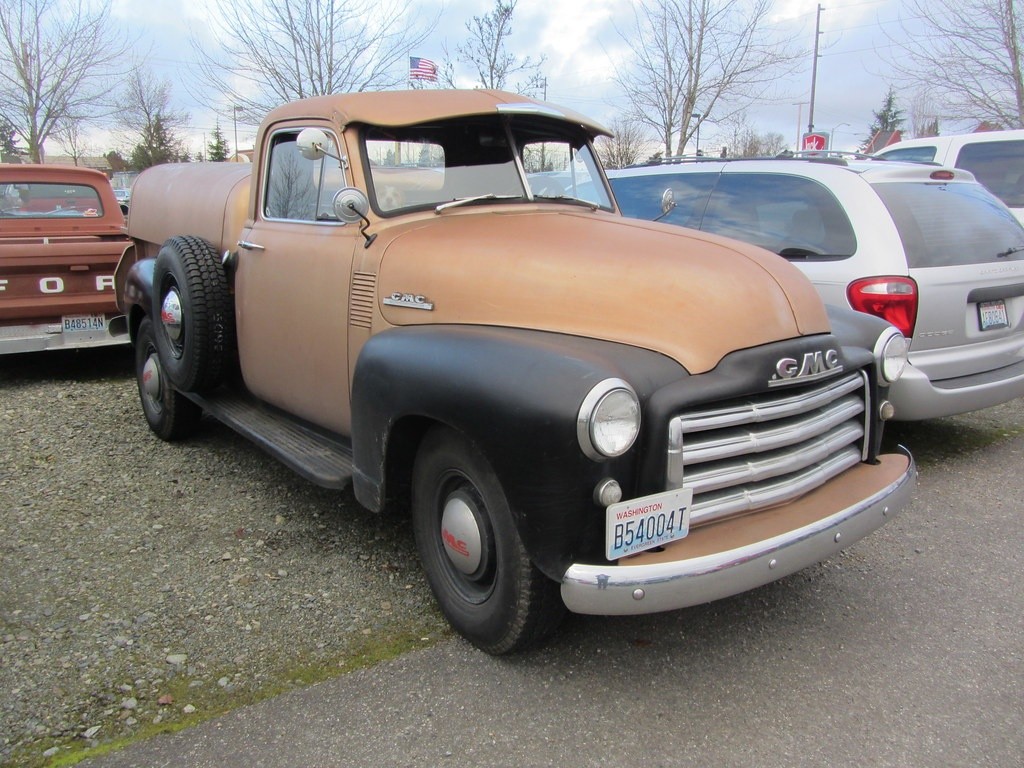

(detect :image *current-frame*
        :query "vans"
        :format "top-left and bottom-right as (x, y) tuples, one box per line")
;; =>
(865, 126), (1024, 230)
(524, 155), (1024, 423)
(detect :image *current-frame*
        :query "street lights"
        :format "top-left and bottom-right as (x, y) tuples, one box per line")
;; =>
(789, 100), (809, 157)
(692, 113), (701, 163)
(233, 104), (243, 164)
(830, 123), (851, 157)
(855, 132), (876, 155)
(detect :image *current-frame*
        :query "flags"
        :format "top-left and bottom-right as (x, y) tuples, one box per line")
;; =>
(410, 56), (439, 82)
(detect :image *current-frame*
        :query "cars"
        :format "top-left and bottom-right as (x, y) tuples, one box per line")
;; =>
(105, 88), (917, 657)
(0, 160), (135, 355)
(1, 182), (131, 213)
